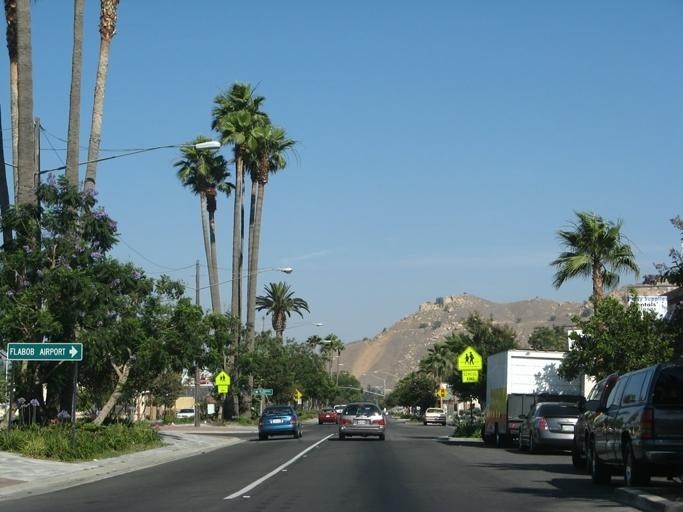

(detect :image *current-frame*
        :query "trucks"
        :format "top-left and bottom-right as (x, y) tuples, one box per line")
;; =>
(481, 349), (598, 446)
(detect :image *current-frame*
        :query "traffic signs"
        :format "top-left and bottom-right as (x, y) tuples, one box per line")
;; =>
(7, 343), (82, 361)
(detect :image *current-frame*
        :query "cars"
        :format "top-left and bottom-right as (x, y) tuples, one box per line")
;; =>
(176, 408), (196, 419)
(258, 405), (303, 440)
(318, 402), (386, 441)
(519, 362), (682, 486)
(422, 407), (446, 425)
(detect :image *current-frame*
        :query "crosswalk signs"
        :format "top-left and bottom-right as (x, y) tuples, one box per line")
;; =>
(215, 370), (231, 385)
(458, 345), (482, 370)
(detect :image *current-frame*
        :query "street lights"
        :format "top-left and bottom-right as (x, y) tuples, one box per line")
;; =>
(324, 355), (343, 371)
(193, 260), (292, 426)
(34, 111), (220, 261)
(362, 369), (398, 395)
(260, 315), (324, 334)
